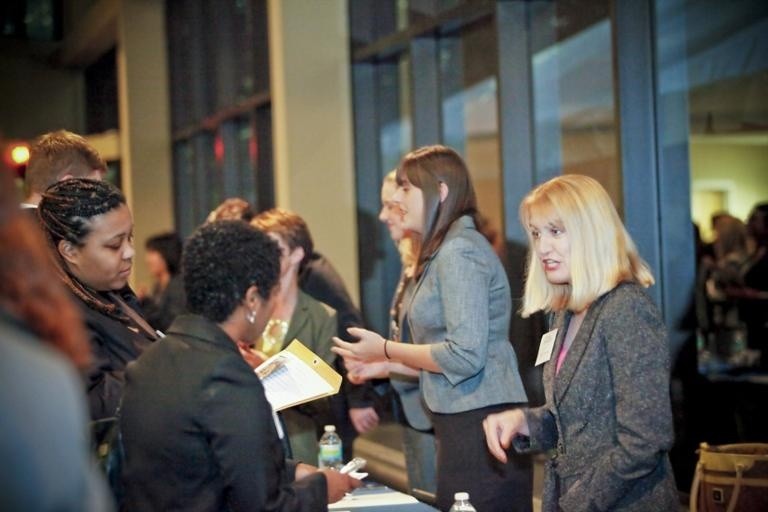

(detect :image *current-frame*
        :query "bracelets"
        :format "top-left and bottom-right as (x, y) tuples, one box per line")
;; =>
(382, 341), (390, 363)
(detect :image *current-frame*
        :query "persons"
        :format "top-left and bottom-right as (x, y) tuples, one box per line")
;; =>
(17, 130), (109, 215)
(330, 145), (532, 510)
(116, 218), (365, 512)
(1, 144), (116, 510)
(248, 209), (341, 467)
(694, 201), (767, 368)
(483, 175), (679, 512)
(37, 179), (156, 439)
(376, 168), (437, 500)
(206, 198), (402, 460)
(144, 234), (186, 318)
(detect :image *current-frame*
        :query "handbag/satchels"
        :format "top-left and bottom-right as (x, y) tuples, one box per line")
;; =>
(690, 443), (767, 512)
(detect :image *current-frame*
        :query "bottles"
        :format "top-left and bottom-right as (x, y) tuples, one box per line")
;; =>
(318, 424), (344, 470)
(448, 492), (477, 512)
